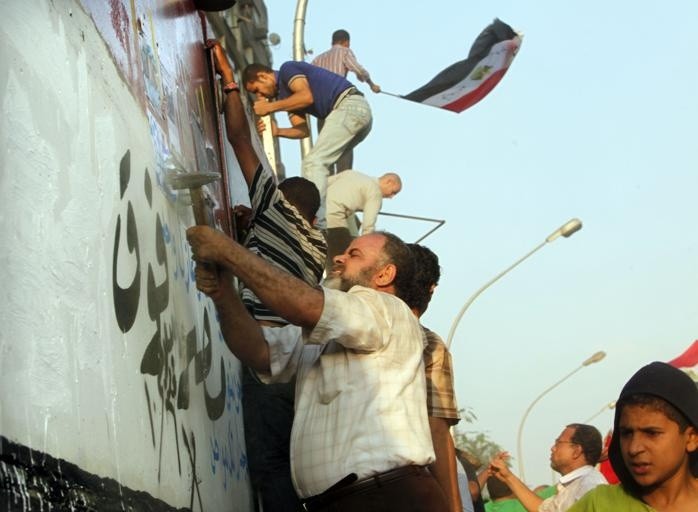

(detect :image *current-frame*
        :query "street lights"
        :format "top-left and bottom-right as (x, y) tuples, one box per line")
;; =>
(516, 351), (607, 482)
(445, 219), (584, 446)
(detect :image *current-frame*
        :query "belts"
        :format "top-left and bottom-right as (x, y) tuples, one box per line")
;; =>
(298, 464), (432, 512)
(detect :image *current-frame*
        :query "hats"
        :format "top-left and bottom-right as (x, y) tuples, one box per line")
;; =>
(608, 362), (698, 488)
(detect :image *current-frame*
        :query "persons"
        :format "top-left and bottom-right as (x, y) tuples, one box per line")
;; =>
(566, 360), (698, 511)
(311, 30), (381, 176)
(402, 243), (508, 511)
(242, 61), (374, 238)
(327, 169), (402, 252)
(186, 225), (451, 512)
(207, 39), (329, 511)
(486, 423), (610, 511)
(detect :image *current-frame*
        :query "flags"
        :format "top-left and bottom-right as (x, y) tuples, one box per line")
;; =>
(401, 18), (525, 113)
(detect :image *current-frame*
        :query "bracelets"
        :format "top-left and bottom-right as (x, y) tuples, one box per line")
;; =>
(223, 81), (240, 95)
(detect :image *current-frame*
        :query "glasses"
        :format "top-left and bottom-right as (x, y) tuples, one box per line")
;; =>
(557, 439), (583, 445)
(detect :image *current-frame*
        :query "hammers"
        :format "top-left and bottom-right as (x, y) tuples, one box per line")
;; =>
(171, 172), (222, 291)
